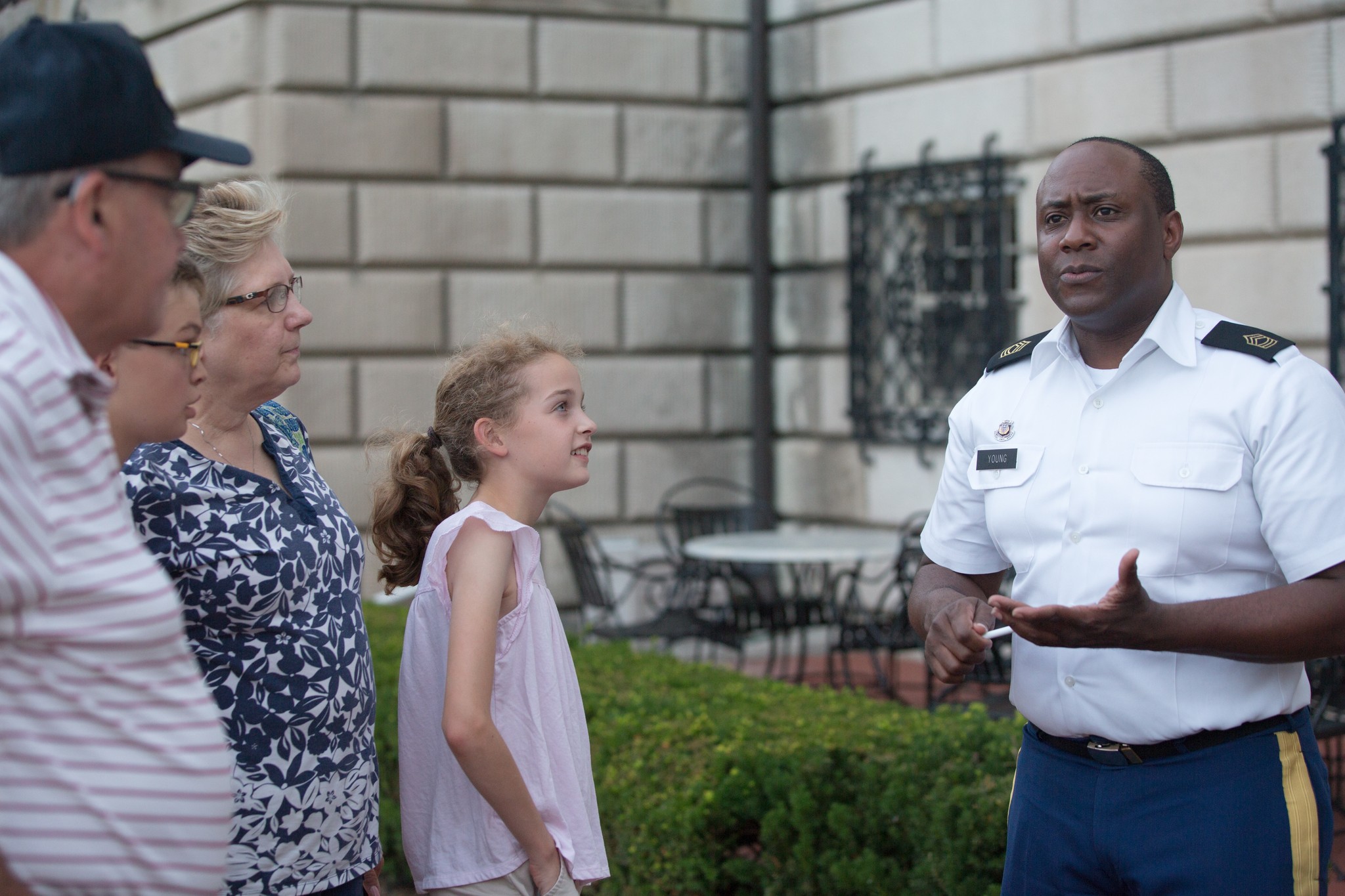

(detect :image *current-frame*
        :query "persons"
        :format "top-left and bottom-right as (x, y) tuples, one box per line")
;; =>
(369, 334), (611, 896)
(906, 136), (1345, 896)
(0, 16), (381, 896)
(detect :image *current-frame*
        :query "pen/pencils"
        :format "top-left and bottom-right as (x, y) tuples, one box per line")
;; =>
(984, 625), (1013, 638)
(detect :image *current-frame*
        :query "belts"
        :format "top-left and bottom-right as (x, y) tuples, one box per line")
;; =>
(1035, 723), (1290, 766)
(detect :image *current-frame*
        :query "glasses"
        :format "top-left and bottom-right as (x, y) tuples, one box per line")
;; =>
(126, 337), (203, 367)
(53, 169), (201, 228)
(219, 277), (304, 313)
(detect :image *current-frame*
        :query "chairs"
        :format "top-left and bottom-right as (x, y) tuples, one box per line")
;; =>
(543, 478), (1014, 713)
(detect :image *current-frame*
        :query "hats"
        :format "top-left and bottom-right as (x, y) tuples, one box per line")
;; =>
(0, 15), (252, 177)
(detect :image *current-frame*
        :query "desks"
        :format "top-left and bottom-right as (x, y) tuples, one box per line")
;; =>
(683, 531), (902, 682)
